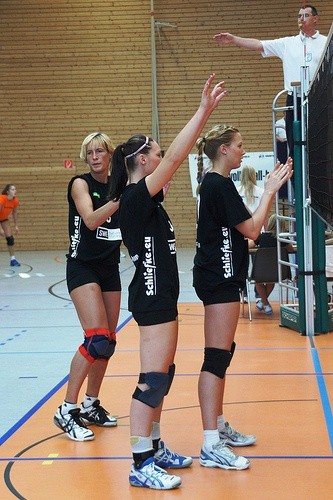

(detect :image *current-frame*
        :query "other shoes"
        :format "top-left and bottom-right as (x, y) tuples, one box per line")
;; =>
(240, 296), (248, 304)
(256, 302), (263, 311)
(10, 260), (20, 266)
(256, 298), (262, 303)
(263, 305), (272, 314)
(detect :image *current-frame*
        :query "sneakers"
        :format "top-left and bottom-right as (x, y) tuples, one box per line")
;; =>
(219, 421), (256, 447)
(129, 457), (181, 490)
(53, 405), (95, 441)
(199, 439), (250, 469)
(154, 440), (192, 468)
(80, 399), (117, 426)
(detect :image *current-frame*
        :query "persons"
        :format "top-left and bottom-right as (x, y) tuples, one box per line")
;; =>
(248, 213), (293, 315)
(234, 165), (265, 304)
(109, 73), (228, 490)
(275, 111), (287, 199)
(54, 132), (169, 441)
(193, 124), (294, 470)
(0, 184), (22, 267)
(213, 6), (329, 189)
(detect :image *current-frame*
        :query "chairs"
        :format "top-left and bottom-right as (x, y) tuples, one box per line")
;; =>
(240, 245), (297, 322)
